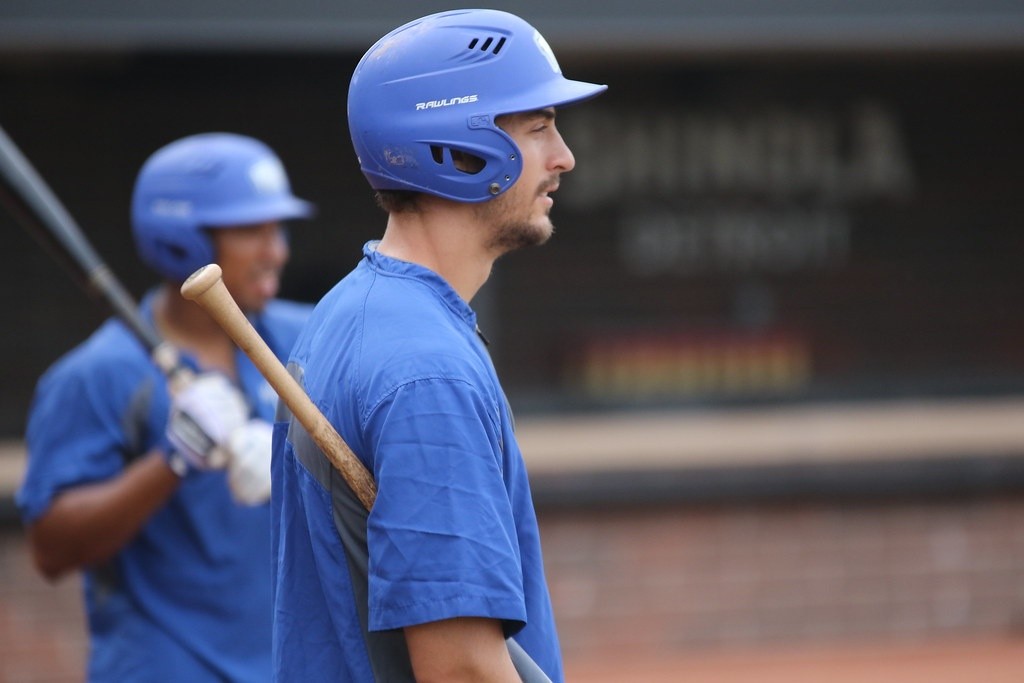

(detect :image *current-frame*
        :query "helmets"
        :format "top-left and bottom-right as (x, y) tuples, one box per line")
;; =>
(345, 6), (610, 202)
(129, 130), (314, 290)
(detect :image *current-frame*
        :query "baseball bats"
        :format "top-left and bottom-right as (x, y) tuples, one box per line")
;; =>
(178, 261), (556, 683)
(0, 126), (197, 397)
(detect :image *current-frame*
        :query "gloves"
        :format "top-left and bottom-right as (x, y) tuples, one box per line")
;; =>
(155, 368), (280, 508)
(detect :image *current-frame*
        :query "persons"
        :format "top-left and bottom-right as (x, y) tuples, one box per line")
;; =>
(15, 133), (315, 683)
(272, 9), (608, 683)
